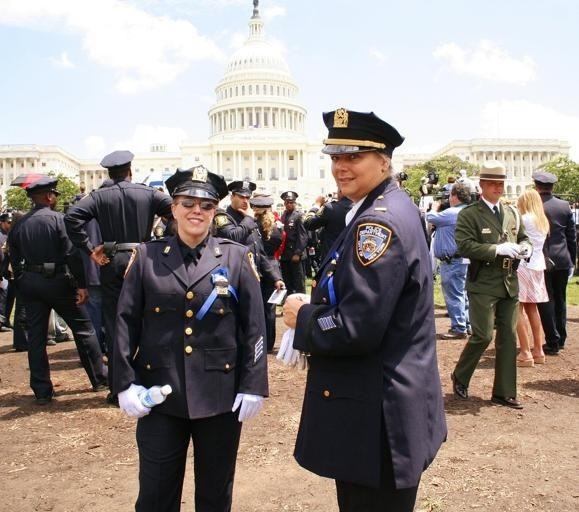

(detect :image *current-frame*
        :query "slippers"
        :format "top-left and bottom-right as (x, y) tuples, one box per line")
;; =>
(516, 356), (545, 367)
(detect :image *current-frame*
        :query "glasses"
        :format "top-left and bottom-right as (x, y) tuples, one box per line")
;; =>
(174, 199), (218, 210)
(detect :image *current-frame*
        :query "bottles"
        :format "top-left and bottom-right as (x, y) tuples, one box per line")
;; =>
(138, 383), (173, 407)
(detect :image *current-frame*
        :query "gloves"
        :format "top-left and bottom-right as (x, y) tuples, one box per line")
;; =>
(117, 383), (153, 419)
(496, 242), (532, 260)
(276, 327), (307, 371)
(232, 390), (263, 423)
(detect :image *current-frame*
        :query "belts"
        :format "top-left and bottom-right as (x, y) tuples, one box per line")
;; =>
(100, 241), (140, 253)
(18, 263), (67, 275)
(440, 257), (460, 261)
(473, 258), (519, 269)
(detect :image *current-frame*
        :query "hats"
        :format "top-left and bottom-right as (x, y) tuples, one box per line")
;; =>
(531, 171), (559, 186)
(321, 108), (406, 155)
(25, 179), (61, 195)
(165, 165), (228, 208)
(227, 180), (274, 207)
(280, 191), (299, 201)
(0, 213), (15, 222)
(100, 150), (135, 169)
(479, 163), (511, 181)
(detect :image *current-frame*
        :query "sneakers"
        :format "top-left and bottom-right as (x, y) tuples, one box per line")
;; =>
(35, 388), (55, 405)
(542, 343), (566, 353)
(92, 382), (109, 392)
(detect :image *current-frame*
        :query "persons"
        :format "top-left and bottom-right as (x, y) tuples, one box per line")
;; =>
(571, 202), (579, 259)
(280, 105), (450, 512)
(104, 162), (269, 512)
(1, 150), (174, 405)
(212, 182), (351, 354)
(530, 170), (577, 355)
(425, 169), (476, 340)
(450, 158), (536, 410)
(515, 187), (553, 369)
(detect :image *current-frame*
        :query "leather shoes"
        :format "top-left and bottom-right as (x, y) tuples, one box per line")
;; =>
(440, 330), (472, 340)
(47, 333), (75, 345)
(492, 395), (523, 411)
(450, 372), (468, 401)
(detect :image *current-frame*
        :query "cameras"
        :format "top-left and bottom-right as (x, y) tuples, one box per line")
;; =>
(397, 172), (408, 180)
(325, 193), (334, 199)
(518, 244), (528, 255)
